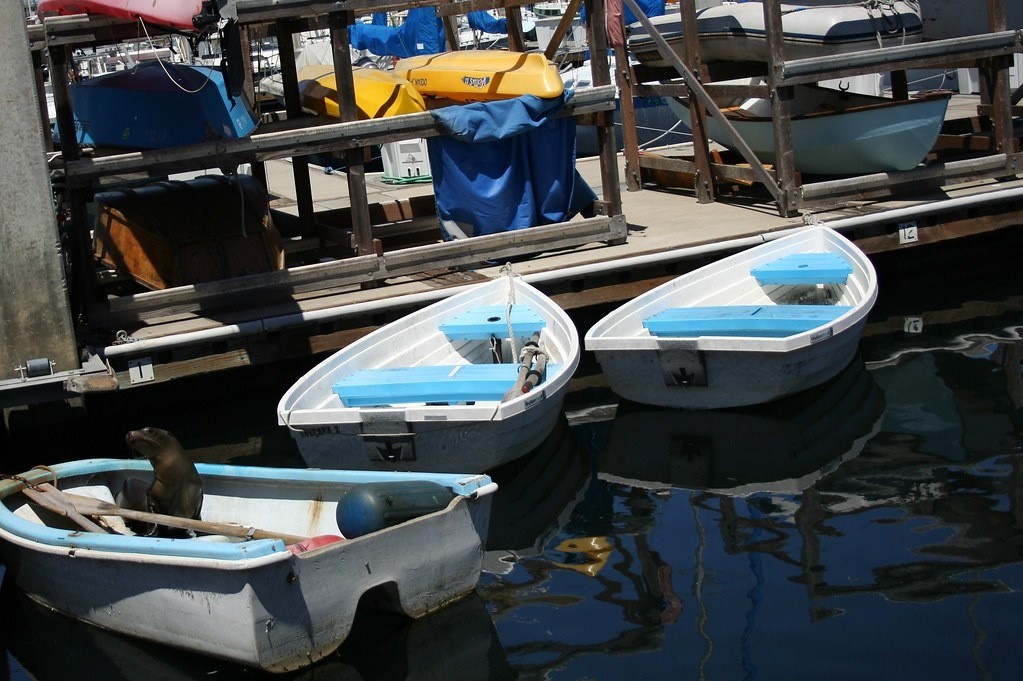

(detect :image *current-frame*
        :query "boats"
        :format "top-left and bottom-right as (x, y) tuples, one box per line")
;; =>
(584, 223), (880, 408)
(1, 460), (500, 676)
(53, 62), (260, 148)
(0, 568), (505, 681)
(660, 81), (960, 178)
(596, 359), (889, 502)
(290, 59), (426, 121)
(33, 0), (234, 40)
(477, 388), (600, 573)
(91, 176), (291, 294)
(276, 260), (582, 476)
(392, 51), (561, 102)
(630, 0), (924, 73)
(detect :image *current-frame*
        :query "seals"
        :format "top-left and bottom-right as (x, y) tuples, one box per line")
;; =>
(125, 426), (203, 541)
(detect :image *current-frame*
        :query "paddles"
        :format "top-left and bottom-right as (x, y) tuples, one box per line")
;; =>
(503, 331), (539, 403)
(24, 483), (108, 532)
(64, 493), (305, 544)
